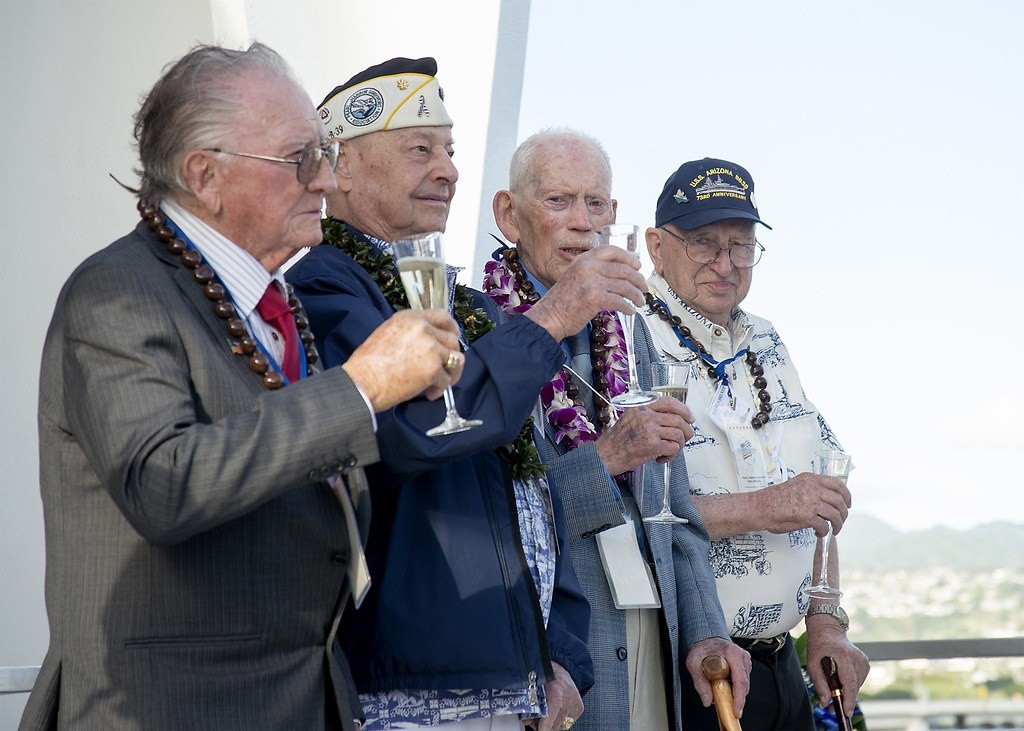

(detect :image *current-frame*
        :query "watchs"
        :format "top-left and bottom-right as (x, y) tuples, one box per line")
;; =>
(806, 604), (850, 631)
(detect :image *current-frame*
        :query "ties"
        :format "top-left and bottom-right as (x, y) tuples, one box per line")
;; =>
(257, 283), (302, 386)
(566, 326), (598, 425)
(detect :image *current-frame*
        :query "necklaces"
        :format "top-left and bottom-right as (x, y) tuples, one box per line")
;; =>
(643, 289), (773, 430)
(306, 217), (552, 485)
(134, 200), (359, 482)
(485, 247), (632, 483)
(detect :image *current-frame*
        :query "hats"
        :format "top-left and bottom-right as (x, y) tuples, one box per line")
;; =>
(315, 57), (452, 141)
(655, 159), (773, 232)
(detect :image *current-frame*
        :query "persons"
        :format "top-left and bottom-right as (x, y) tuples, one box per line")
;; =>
(18, 37), (466, 731)
(645, 158), (872, 731)
(285, 56), (648, 731)
(483, 129), (752, 731)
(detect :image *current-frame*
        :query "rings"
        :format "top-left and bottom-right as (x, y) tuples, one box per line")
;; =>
(446, 349), (460, 372)
(561, 716), (575, 730)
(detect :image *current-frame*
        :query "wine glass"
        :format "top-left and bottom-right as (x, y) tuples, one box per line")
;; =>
(642, 362), (690, 524)
(801, 450), (850, 600)
(391, 231), (483, 436)
(603, 224), (659, 408)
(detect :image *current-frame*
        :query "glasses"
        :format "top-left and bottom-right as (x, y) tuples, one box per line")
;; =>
(200, 137), (339, 185)
(661, 224), (764, 269)
